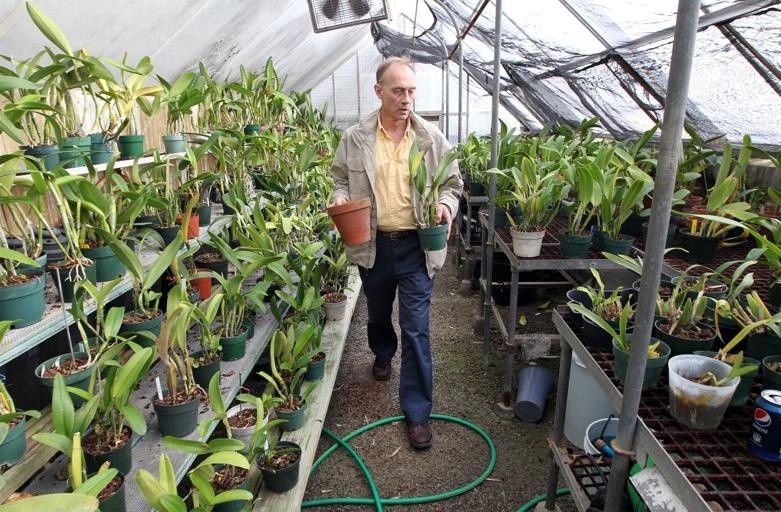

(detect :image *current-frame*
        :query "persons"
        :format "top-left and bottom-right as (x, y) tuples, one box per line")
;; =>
(327, 56), (465, 450)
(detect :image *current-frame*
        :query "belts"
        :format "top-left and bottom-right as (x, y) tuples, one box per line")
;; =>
(374, 229), (419, 240)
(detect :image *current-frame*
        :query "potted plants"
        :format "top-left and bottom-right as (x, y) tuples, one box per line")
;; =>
(405, 139), (462, 251)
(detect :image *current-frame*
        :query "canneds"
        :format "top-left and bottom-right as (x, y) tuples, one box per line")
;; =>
(747, 389), (781, 462)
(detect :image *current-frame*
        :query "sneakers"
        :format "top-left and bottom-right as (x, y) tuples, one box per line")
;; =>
(406, 416), (433, 449)
(372, 354), (392, 381)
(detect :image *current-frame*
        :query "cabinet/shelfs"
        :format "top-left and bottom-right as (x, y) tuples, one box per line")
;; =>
(544, 305), (781, 512)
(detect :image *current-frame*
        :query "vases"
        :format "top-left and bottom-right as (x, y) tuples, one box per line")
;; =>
(328, 197), (372, 247)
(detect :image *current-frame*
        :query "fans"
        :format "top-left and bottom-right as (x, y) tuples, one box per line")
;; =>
(307, 0), (387, 33)
